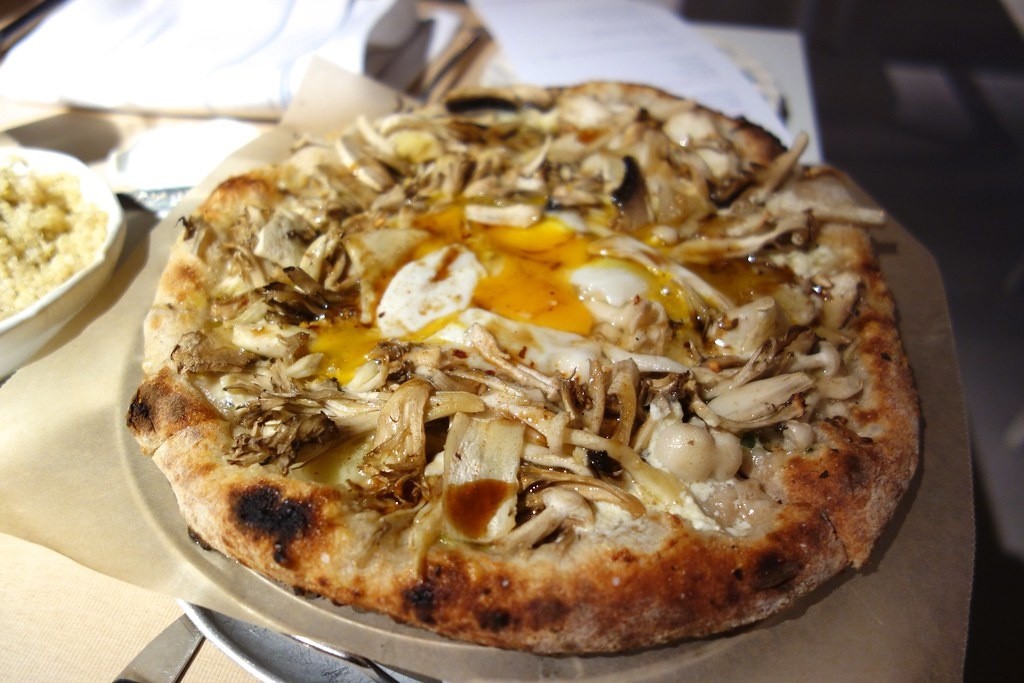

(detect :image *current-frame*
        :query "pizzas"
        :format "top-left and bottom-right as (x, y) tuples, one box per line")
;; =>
(124, 80), (925, 656)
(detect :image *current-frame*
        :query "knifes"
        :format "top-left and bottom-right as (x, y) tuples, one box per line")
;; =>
(112, 613), (205, 683)
(175, 597), (385, 683)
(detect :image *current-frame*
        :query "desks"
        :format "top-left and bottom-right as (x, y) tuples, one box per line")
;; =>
(0, 19), (827, 683)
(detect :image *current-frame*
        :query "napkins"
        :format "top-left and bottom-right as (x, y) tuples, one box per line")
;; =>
(0, 0), (806, 191)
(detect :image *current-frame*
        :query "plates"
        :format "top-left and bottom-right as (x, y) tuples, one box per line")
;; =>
(116, 306), (503, 652)
(0, 146), (125, 355)
